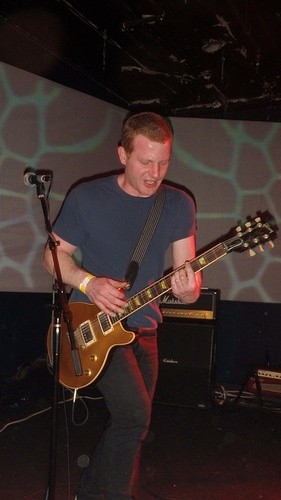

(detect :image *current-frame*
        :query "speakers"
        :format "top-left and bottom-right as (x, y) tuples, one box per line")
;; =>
(153, 316), (217, 409)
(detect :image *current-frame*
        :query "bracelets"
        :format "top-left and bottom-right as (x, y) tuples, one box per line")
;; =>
(78, 273), (95, 294)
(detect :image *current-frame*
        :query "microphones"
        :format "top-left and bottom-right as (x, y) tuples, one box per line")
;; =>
(23, 172), (50, 188)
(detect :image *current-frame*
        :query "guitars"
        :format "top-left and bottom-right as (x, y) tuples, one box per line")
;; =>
(48, 214), (279, 390)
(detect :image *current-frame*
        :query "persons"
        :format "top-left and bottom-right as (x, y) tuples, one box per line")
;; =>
(42, 112), (203, 500)
